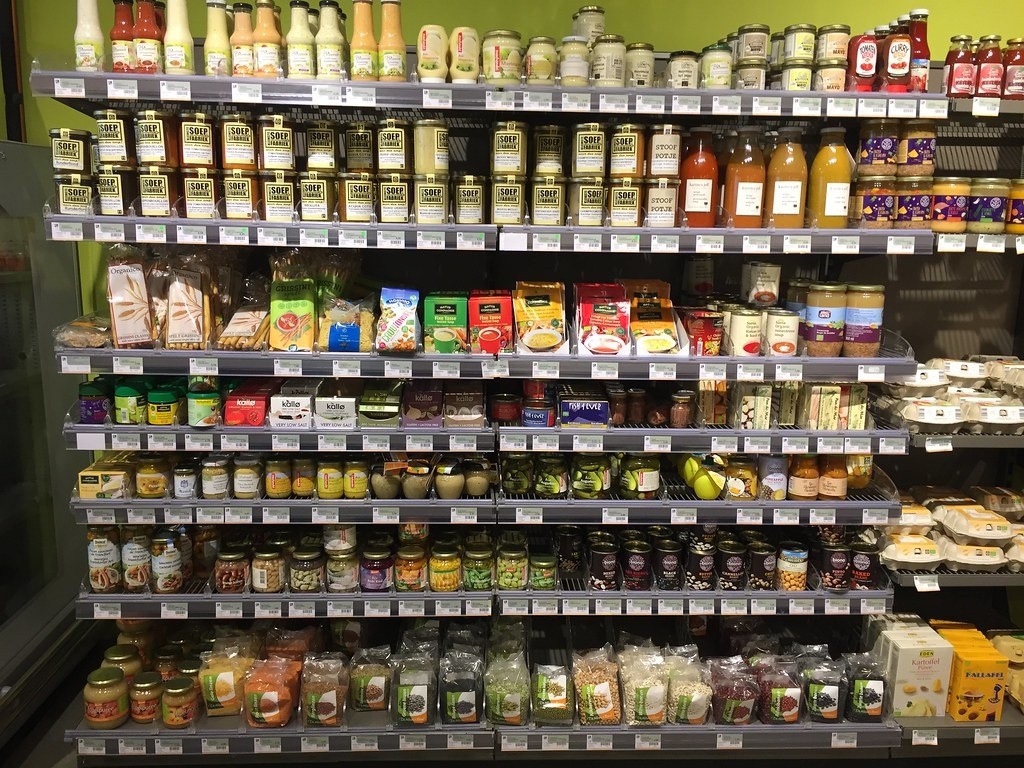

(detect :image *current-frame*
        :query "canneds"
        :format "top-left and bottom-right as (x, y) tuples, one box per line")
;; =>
(45, 105), (1024, 729)
(480, 3), (850, 92)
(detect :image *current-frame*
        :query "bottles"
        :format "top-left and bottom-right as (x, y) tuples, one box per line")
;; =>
(73, 0), (406, 86)
(449, 25), (481, 86)
(679, 123), (851, 229)
(414, 25), (449, 86)
(849, 5), (1024, 101)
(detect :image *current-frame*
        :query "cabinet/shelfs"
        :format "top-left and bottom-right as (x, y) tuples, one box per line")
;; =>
(27, 71), (1024, 767)
